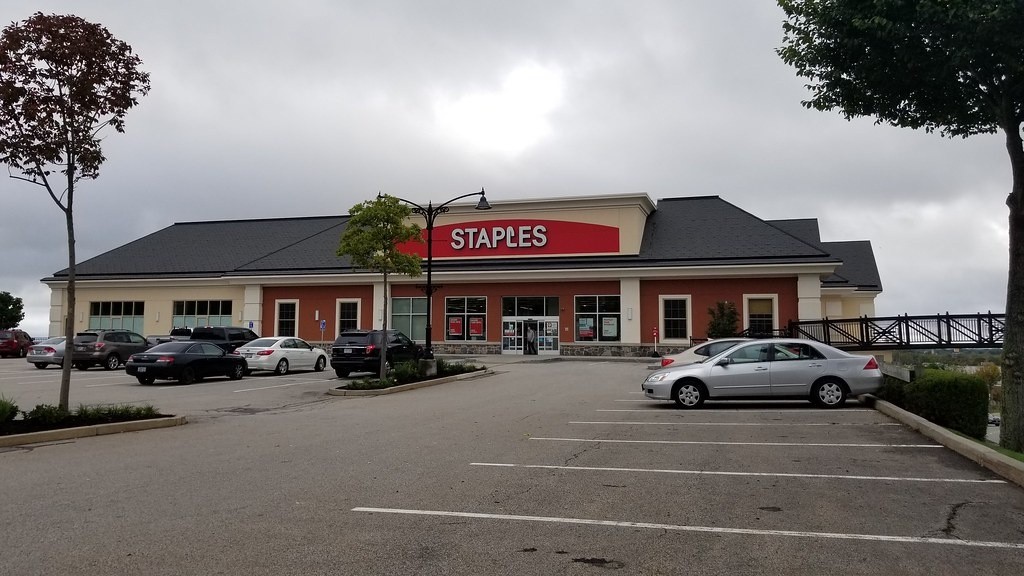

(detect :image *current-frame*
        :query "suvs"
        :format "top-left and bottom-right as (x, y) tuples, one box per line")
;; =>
(330, 328), (433, 378)
(0, 329), (35, 357)
(72, 329), (154, 370)
(191, 324), (260, 353)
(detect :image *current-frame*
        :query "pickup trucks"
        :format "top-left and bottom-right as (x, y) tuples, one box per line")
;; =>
(146, 327), (194, 346)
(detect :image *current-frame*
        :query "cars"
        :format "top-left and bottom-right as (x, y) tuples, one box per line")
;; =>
(234, 336), (329, 375)
(125, 340), (249, 385)
(26, 337), (67, 369)
(661, 338), (809, 369)
(988, 413), (1000, 426)
(642, 338), (884, 409)
(503, 337), (521, 350)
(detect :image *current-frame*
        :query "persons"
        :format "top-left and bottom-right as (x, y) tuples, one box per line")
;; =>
(527, 327), (536, 355)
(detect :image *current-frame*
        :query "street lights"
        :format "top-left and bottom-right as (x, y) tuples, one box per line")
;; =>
(379, 190), (494, 359)
